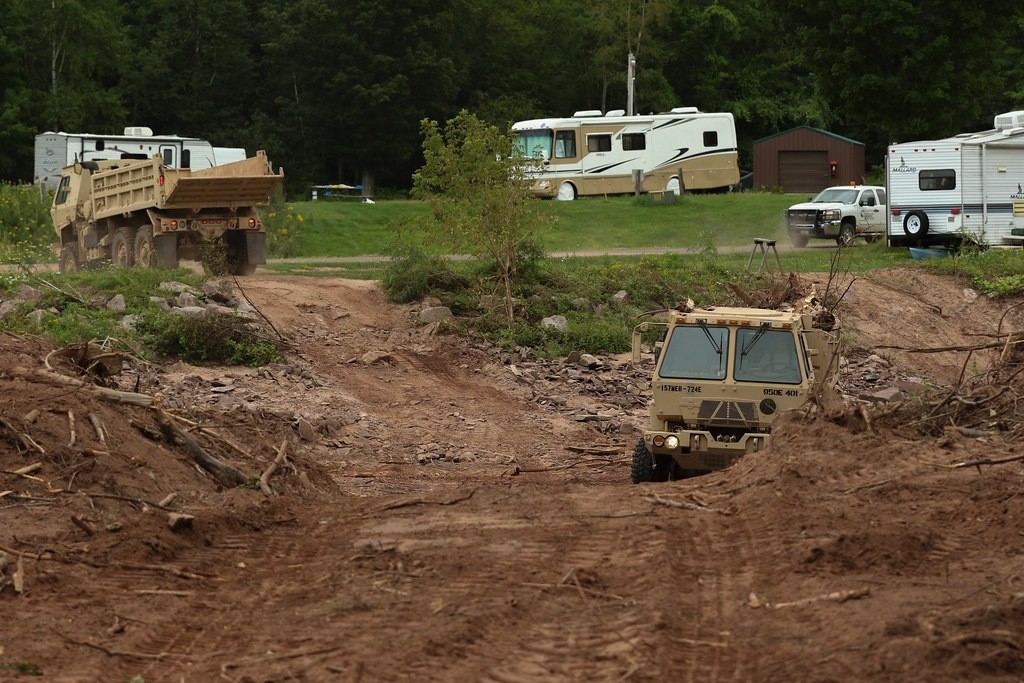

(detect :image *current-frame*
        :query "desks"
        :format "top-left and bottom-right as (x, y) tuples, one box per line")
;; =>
(311, 185), (363, 203)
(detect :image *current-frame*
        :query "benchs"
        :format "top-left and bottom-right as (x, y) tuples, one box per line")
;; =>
(326, 194), (374, 198)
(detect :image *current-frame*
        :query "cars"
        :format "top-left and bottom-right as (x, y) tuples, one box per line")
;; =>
(784, 179), (885, 250)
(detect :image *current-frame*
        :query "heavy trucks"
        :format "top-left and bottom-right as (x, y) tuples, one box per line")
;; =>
(39, 147), (286, 276)
(628, 298), (845, 484)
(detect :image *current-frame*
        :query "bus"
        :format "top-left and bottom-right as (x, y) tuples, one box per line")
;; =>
(505, 104), (742, 202)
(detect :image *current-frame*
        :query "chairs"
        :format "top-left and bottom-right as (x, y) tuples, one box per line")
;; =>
(760, 341), (799, 373)
